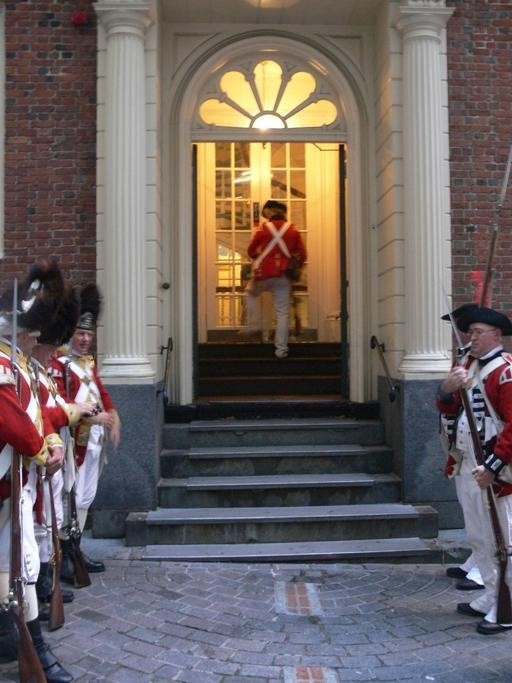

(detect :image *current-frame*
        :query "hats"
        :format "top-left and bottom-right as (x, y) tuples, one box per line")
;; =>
(0, 262), (103, 348)
(261, 197), (286, 216)
(440, 301), (512, 340)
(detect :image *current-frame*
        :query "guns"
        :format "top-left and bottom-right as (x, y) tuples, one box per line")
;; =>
(10, 278), (45, 681)
(33, 364), (64, 631)
(65, 360), (90, 590)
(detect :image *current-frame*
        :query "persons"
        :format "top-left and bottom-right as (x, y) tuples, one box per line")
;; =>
(0, 257), (122, 683)
(438, 302), (488, 589)
(233, 200), (307, 358)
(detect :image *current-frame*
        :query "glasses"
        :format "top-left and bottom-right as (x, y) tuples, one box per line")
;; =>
(467, 327), (496, 337)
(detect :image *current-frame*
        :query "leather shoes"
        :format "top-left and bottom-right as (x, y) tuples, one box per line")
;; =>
(446, 567), (470, 578)
(456, 599), (487, 618)
(477, 617), (511, 635)
(456, 579), (488, 591)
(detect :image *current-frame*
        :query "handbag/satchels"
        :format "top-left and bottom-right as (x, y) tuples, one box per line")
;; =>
(284, 253), (302, 282)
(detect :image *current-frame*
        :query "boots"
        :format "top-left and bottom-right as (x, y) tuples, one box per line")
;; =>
(36, 526), (104, 602)
(0, 604), (21, 665)
(24, 615), (74, 683)
